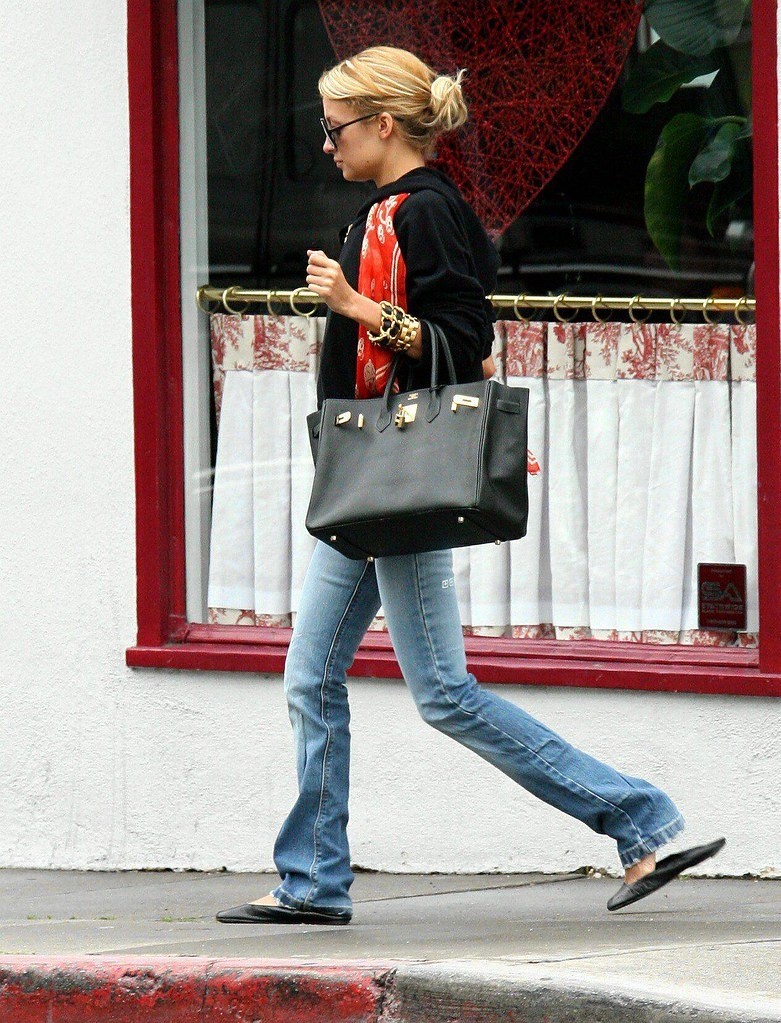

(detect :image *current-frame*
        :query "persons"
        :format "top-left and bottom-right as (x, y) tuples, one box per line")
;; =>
(217, 46), (727, 926)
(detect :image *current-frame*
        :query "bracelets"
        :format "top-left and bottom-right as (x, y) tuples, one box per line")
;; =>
(367, 301), (419, 352)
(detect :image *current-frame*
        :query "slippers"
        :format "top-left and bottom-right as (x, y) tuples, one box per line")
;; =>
(216, 902), (352, 925)
(607, 836), (726, 911)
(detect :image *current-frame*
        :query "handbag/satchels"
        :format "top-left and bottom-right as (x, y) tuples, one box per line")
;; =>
(305, 318), (529, 562)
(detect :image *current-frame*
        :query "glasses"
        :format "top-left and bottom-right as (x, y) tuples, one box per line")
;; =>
(319, 112), (405, 148)
